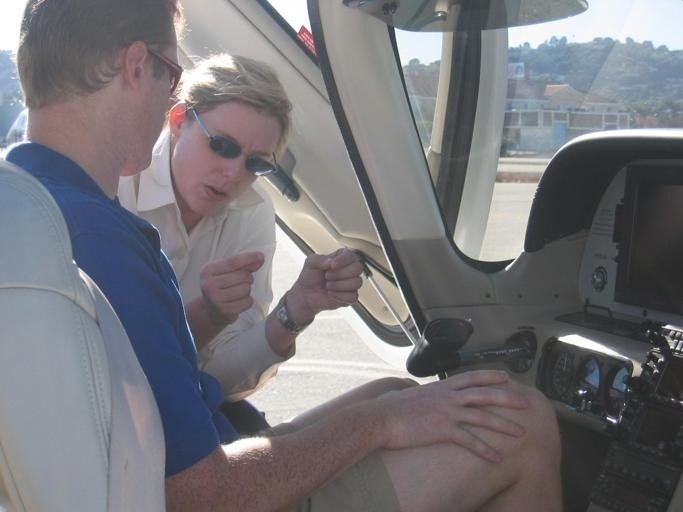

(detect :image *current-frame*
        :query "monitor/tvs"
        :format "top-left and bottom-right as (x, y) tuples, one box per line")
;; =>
(613, 164), (683, 316)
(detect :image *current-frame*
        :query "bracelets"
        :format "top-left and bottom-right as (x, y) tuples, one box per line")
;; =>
(273, 292), (316, 334)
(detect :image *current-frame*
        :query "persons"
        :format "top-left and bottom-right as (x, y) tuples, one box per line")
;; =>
(116, 52), (366, 405)
(4, 0), (566, 511)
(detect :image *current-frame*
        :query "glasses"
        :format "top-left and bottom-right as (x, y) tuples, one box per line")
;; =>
(129, 38), (184, 95)
(191, 107), (279, 179)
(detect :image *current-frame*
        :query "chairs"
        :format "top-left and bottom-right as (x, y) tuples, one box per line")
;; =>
(0, 160), (165, 511)
